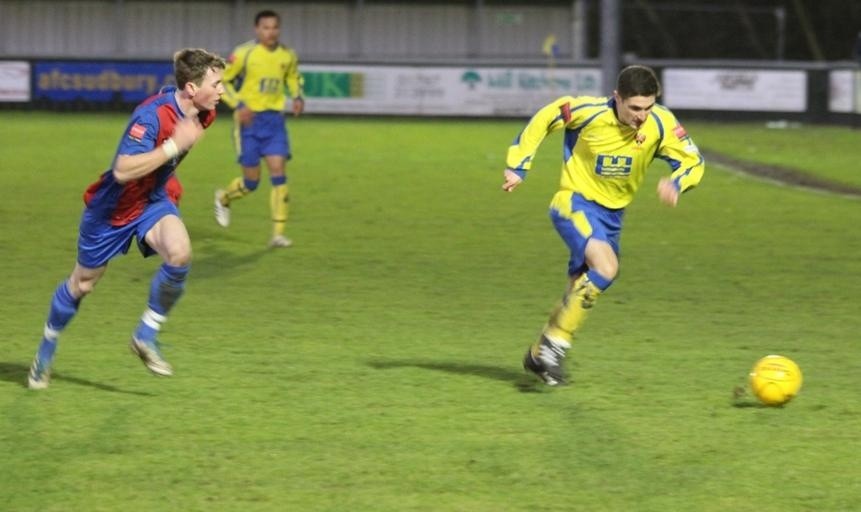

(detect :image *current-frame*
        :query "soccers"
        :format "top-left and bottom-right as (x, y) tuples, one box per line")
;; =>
(750, 355), (804, 407)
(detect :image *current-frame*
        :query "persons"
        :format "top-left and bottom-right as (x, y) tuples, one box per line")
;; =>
(209, 8), (308, 251)
(502, 59), (708, 392)
(22, 45), (230, 396)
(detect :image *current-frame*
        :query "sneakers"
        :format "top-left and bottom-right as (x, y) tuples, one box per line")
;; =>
(128, 331), (174, 377)
(523, 342), (573, 383)
(536, 333), (570, 387)
(268, 233), (293, 246)
(214, 187), (232, 227)
(28, 349), (54, 389)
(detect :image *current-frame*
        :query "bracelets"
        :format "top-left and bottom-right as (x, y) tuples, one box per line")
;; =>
(160, 137), (181, 159)
